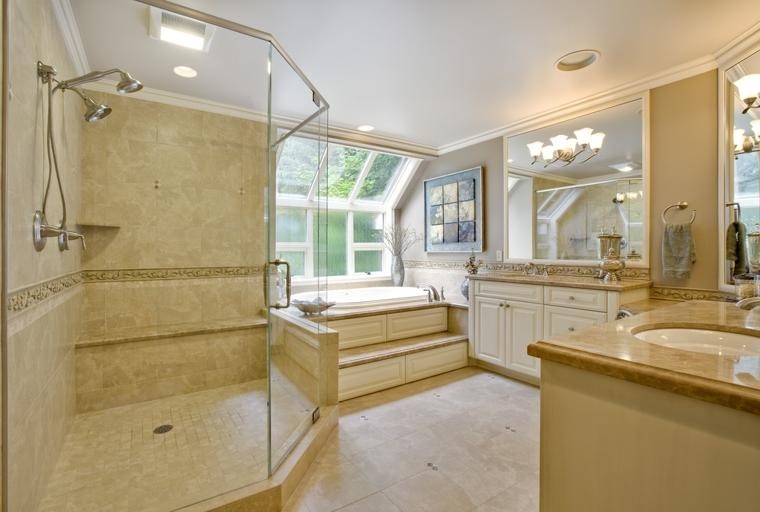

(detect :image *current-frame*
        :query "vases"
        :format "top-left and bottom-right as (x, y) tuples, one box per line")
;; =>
(391, 256), (403, 286)
(461, 276), (470, 302)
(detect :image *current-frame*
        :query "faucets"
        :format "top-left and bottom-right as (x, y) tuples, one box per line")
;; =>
(525, 262), (539, 275)
(735, 298), (760, 310)
(428, 284), (440, 301)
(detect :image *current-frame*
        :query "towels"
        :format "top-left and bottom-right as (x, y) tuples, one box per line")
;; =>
(662, 223), (696, 283)
(727, 222), (749, 277)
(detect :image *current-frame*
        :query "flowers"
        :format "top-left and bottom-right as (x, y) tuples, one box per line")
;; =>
(462, 254), (484, 275)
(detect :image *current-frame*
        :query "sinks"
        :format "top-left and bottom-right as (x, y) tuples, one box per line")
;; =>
(631, 321), (760, 357)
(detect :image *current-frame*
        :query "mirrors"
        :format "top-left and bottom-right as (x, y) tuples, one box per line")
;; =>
(717, 43), (760, 295)
(502, 90), (651, 269)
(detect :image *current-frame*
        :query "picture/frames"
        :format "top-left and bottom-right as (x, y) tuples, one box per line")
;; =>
(423, 166), (485, 253)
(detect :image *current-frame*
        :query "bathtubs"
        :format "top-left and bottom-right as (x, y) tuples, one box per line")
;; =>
(290, 286), (429, 307)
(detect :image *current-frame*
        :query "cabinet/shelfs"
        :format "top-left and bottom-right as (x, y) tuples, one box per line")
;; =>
(467, 278), (543, 389)
(543, 282), (650, 341)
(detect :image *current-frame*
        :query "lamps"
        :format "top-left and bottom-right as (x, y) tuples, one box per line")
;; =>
(733, 119), (760, 160)
(732, 72), (760, 114)
(526, 127), (606, 170)
(612, 180), (643, 204)
(608, 161), (641, 173)
